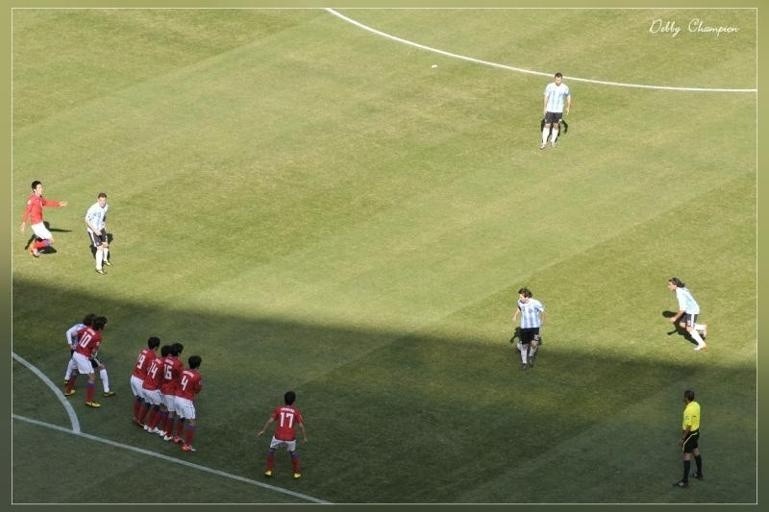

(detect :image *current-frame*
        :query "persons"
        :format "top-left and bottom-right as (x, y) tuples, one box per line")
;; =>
(20, 181), (68, 257)
(173, 355), (203, 451)
(540, 73), (571, 148)
(85, 193), (113, 275)
(143, 345), (170, 432)
(159, 342), (184, 441)
(66, 313), (115, 397)
(64, 316), (108, 408)
(130, 336), (160, 428)
(673, 390), (703, 486)
(668, 277), (707, 351)
(258, 391), (307, 479)
(512, 287), (546, 368)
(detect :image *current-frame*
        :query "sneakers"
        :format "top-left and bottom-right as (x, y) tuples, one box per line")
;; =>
(103, 260), (111, 266)
(521, 355), (534, 371)
(540, 140), (555, 150)
(31, 244), (40, 256)
(701, 324), (707, 340)
(691, 470), (703, 480)
(694, 342), (706, 352)
(671, 480), (688, 487)
(63, 379), (77, 396)
(95, 267), (106, 276)
(85, 400), (101, 409)
(103, 390), (116, 398)
(263, 469), (273, 477)
(132, 416), (196, 453)
(292, 472), (302, 478)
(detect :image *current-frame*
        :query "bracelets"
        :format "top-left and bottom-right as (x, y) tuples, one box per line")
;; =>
(260, 430), (264, 434)
(680, 437), (685, 440)
